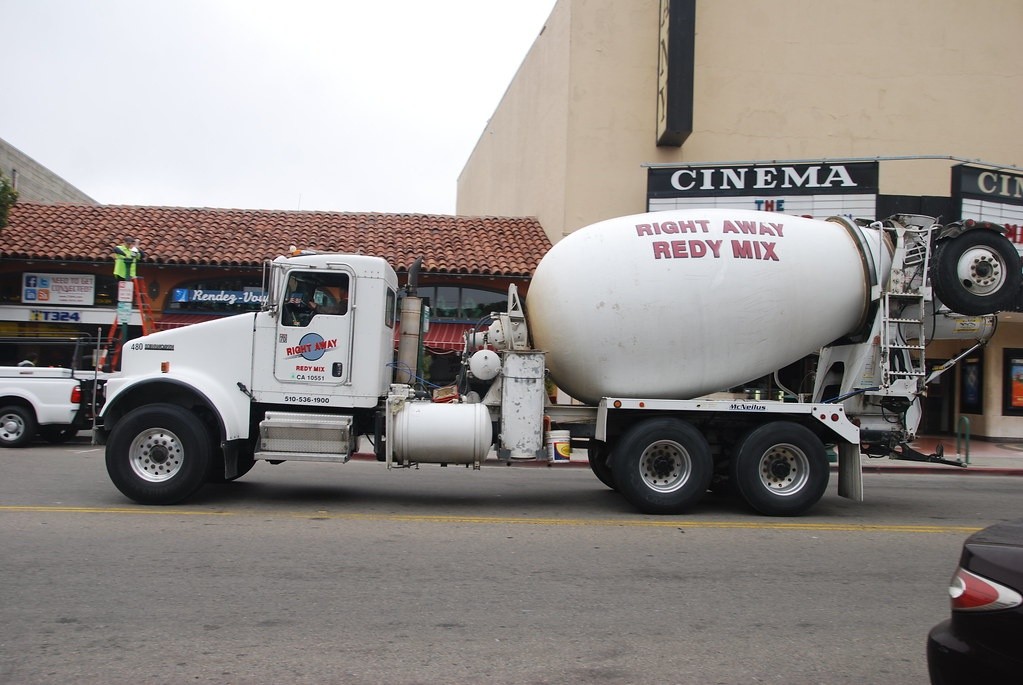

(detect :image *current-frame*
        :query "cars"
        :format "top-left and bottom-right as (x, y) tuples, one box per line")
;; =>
(925, 521), (1023, 685)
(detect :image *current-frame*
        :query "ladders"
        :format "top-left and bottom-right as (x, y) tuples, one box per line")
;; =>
(97, 275), (156, 371)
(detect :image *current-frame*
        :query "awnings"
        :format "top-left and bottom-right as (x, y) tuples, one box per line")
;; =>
(153, 314), (223, 331)
(423, 321), (503, 354)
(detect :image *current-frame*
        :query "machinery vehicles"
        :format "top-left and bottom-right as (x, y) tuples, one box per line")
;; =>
(95, 209), (1023, 518)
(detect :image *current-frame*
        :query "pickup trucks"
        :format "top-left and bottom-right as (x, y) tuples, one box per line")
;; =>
(0, 327), (121, 448)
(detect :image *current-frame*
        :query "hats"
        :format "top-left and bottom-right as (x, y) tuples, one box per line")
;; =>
(125, 236), (135, 243)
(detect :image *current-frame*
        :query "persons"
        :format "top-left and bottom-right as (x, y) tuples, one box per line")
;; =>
(113, 237), (144, 283)
(308, 282), (347, 316)
